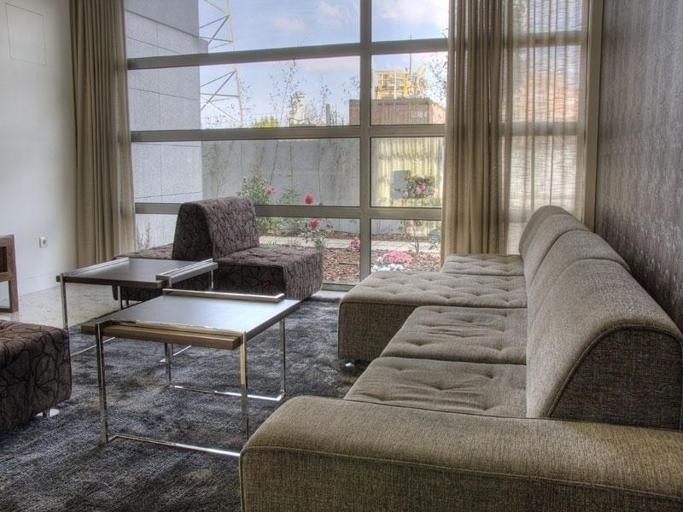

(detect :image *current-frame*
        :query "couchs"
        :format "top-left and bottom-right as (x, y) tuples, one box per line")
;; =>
(237, 205), (683, 511)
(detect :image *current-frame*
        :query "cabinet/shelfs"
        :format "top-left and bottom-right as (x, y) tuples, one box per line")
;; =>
(0, 234), (19, 311)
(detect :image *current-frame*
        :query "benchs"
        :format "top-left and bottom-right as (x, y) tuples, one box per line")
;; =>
(112, 194), (323, 302)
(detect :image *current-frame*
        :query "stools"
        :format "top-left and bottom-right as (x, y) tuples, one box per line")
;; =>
(0, 319), (72, 435)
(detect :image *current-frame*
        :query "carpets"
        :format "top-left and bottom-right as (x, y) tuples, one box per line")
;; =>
(0, 297), (373, 511)
